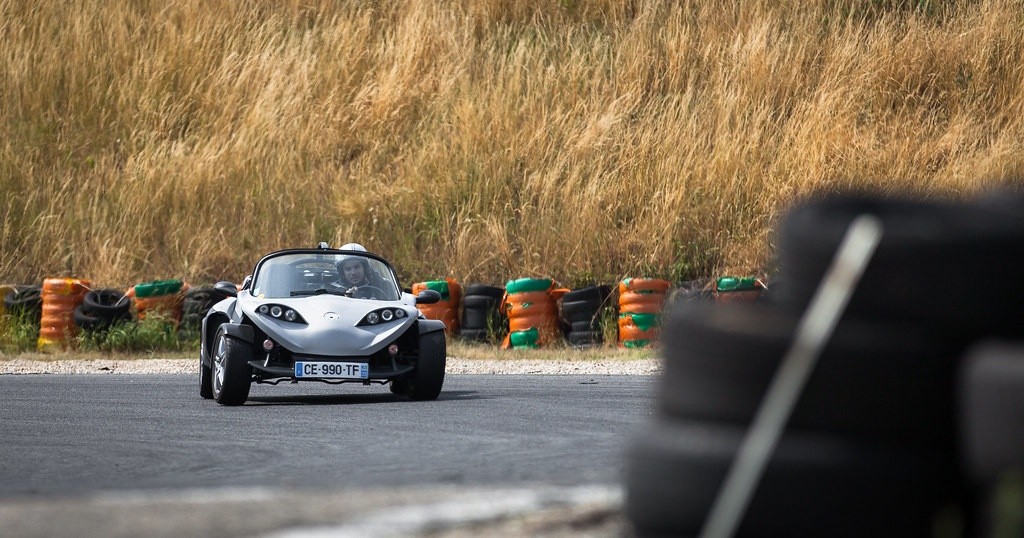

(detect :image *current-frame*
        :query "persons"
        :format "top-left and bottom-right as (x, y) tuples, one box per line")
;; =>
(325, 244), (397, 302)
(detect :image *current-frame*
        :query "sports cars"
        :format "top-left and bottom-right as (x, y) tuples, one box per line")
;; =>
(199, 243), (447, 406)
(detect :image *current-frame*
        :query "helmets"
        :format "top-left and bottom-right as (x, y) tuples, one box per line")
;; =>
(335, 243), (370, 276)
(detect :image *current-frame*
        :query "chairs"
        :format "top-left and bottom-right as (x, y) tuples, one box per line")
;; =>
(261, 264), (305, 297)
